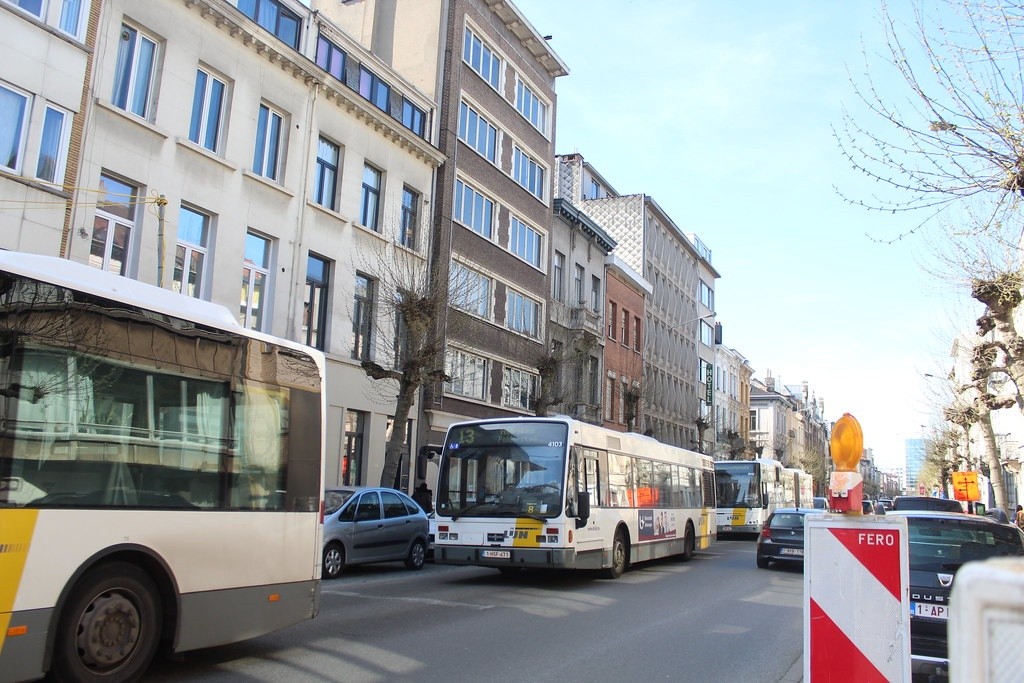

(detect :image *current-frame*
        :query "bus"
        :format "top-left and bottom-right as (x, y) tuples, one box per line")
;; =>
(712, 458), (814, 534)
(0, 249), (331, 683)
(417, 414), (718, 582)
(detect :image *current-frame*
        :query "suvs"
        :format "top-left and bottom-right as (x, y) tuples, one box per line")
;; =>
(894, 496), (963, 512)
(26, 486), (203, 509)
(894, 510), (1024, 683)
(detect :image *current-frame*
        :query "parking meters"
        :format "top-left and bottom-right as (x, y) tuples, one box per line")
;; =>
(801, 412), (913, 683)
(973, 502), (987, 544)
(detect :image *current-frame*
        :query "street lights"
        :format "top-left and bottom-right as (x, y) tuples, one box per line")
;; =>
(925, 372), (974, 515)
(639, 310), (717, 440)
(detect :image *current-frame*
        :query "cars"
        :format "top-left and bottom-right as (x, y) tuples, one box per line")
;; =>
(878, 500), (894, 510)
(322, 487), (430, 579)
(863, 499), (875, 515)
(426, 497), (498, 560)
(873, 502), (886, 514)
(813, 497), (830, 510)
(756, 508), (827, 573)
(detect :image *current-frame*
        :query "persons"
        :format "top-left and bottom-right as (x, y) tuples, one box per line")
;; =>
(1016, 505), (1024, 532)
(408, 482), (434, 514)
